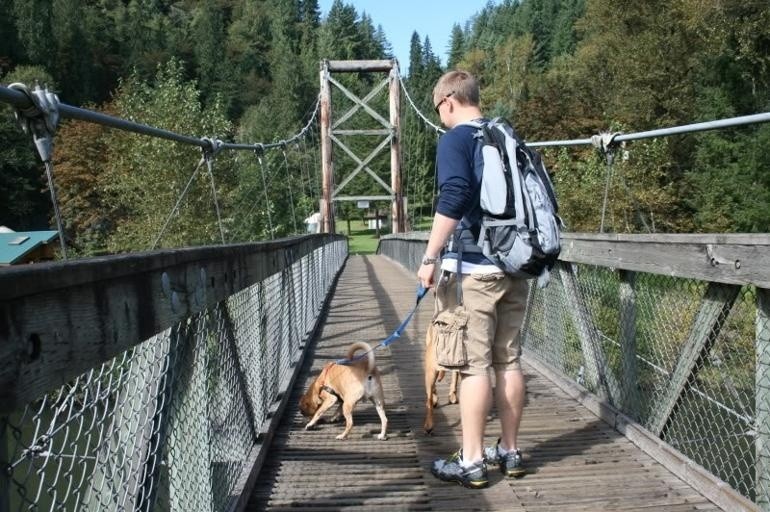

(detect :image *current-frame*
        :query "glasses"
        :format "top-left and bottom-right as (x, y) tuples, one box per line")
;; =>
(433, 91), (456, 114)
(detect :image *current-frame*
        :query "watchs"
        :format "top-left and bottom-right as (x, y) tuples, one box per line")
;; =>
(418, 255), (437, 268)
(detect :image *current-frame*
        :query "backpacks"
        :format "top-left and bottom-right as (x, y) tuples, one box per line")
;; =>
(453, 117), (561, 279)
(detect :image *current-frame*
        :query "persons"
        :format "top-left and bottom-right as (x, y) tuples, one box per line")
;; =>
(414, 68), (542, 491)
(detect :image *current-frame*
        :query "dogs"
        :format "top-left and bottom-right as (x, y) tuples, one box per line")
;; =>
(424, 291), (459, 437)
(297, 341), (390, 440)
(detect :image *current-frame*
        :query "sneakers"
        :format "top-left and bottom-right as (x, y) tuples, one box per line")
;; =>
(483, 439), (523, 477)
(432, 447), (489, 489)
